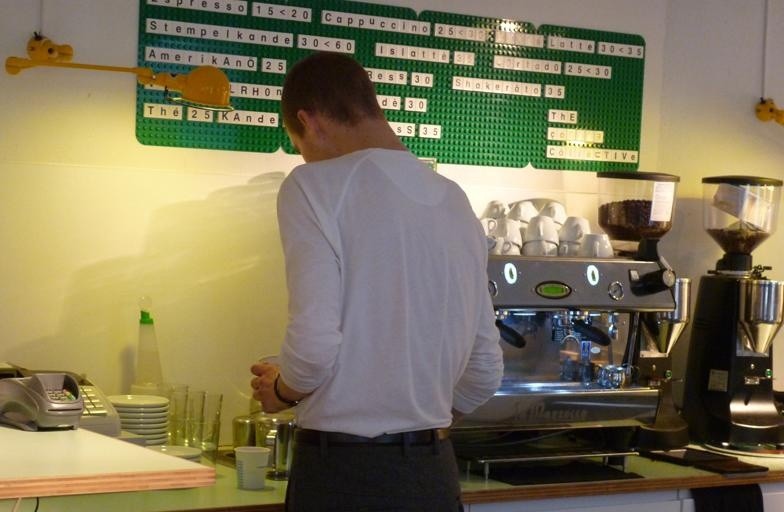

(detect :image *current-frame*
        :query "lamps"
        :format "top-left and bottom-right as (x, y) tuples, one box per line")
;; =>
(6, 33), (235, 113)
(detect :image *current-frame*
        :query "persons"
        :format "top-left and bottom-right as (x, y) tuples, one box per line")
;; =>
(251, 51), (505, 511)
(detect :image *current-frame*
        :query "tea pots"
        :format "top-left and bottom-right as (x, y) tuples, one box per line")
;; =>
(595, 363), (635, 390)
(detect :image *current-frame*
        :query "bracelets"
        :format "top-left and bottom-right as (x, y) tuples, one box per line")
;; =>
(273, 371), (304, 407)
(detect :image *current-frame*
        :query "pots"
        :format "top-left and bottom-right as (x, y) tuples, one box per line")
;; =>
(230, 410), (298, 478)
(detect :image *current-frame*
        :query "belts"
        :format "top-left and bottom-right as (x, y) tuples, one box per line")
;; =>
(297, 427), (451, 445)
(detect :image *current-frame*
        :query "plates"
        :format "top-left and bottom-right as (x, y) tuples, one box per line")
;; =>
(107, 394), (202, 464)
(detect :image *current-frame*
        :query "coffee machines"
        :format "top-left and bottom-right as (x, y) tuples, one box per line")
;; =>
(683, 174), (784, 445)
(596, 170), (692, 450)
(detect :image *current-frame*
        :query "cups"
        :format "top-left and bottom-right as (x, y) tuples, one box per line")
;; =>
(233, 446), (272, 491)
(157, 382), (224, 461)
(481, 199), (614, 259)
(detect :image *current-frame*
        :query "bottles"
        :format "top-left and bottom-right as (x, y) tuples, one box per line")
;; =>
(129, 310), (165, 393)
